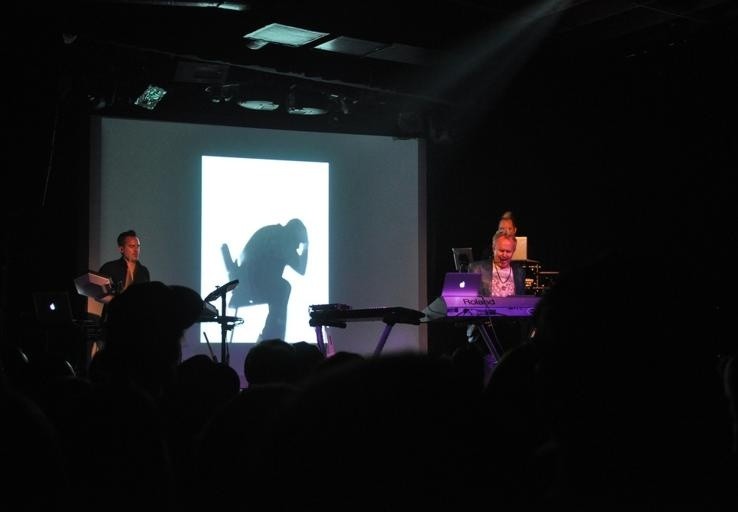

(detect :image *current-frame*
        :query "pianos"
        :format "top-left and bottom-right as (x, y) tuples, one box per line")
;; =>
(444, 297), (540, 316)
(311, 306), (426, 320)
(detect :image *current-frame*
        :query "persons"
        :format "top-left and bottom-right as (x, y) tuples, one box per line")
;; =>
(465, 227), (529, 348)
(92, 229), (150, 322)
(494, 208), (518, 237)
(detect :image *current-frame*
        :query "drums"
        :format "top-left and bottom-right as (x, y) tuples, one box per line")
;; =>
(509, 258), (544, 290)
(540, 270), (559, 289)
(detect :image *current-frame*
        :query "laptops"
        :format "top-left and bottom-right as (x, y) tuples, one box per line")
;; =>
(441, 272), (481, 297)
